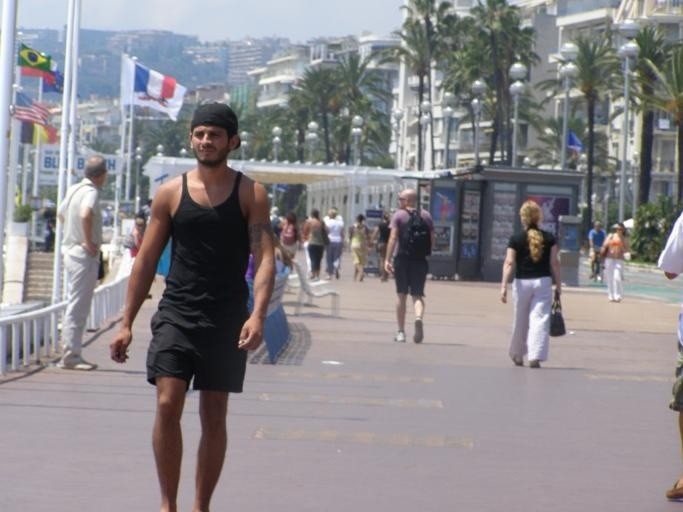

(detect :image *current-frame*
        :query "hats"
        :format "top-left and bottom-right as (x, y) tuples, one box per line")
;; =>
(191, 102), (239, 149)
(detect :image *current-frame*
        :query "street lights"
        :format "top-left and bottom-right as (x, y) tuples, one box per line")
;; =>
(612, 19), (642, 228)
(556, 39), (581, 169)
(468, 77), (488, 164)
(507, 62), (526, 169)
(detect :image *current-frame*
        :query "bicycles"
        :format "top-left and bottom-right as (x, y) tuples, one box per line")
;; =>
(588, 252), (605, 285)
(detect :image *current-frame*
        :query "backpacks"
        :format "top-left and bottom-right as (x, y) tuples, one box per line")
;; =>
(399, 207), (430, 256)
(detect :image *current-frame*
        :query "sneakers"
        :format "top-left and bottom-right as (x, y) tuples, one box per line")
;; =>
(60, 360), (96, 370)
(393, 332), (405, 342)
(413, 317), (422, 343)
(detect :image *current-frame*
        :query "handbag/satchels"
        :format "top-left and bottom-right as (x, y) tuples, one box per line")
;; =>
(548, 312), (564, 336)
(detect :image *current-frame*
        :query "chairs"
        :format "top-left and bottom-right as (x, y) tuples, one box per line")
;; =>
(245, 266), (291, 364)
(284, 257), (341, 318)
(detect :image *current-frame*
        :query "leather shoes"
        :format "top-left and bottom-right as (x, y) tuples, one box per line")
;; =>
(666, 480), (682, 499)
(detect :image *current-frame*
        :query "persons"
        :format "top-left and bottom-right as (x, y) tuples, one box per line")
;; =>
(588, 221), (631, 303)
(657, 210), (683, 502)
(130, 199), (152, 298)
(56, 156), (108, 371)
(500, 200), (561, 368)
(269, 206), (392, 283)
(41, 219), (56, 252)
(108, 102), (276, 512)
(384, 189), (435, 342)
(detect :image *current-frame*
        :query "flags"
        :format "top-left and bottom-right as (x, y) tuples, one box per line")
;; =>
(567, 130), (583, 153)
(12, 42), (64, 144)
(120, 53), (188, 123)
